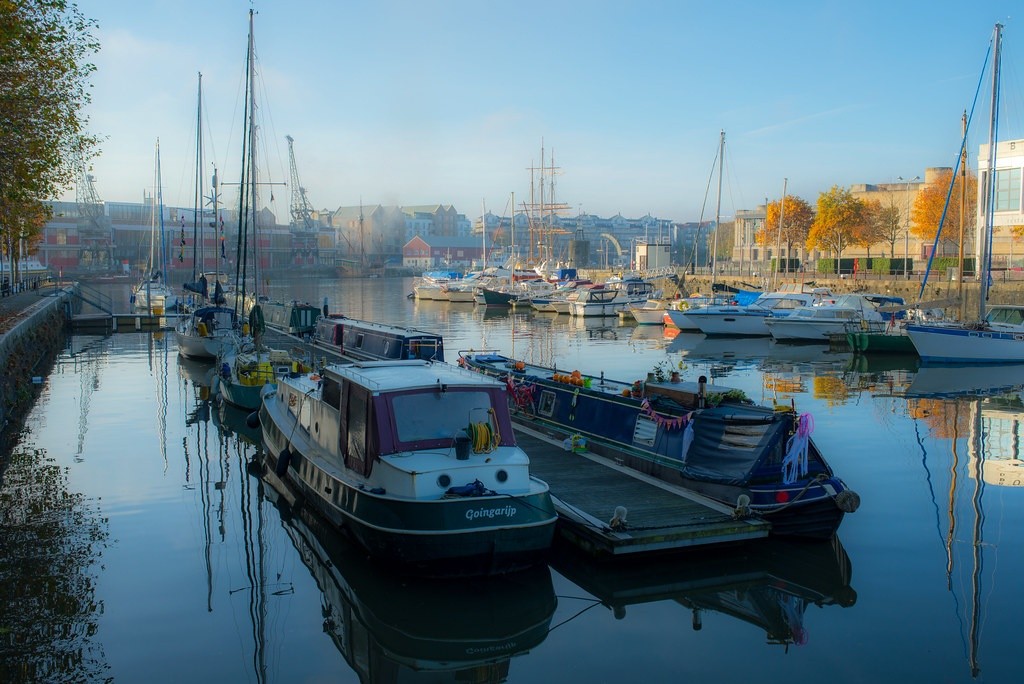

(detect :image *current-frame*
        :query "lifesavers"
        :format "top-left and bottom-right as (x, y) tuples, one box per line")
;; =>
(861, 320), (868, 331)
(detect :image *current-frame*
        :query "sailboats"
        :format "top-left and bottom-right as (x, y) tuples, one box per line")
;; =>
(211, 8), (327, 409)
(905, 24), (1024, 363)
(129, 136), (174, 309)
(336, 198), (385, 278)
(175, 70), (249, 362)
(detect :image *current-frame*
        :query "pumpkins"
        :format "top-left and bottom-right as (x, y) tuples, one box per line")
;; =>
(553, 370), (584, 388)
(516, 361), (524, 369)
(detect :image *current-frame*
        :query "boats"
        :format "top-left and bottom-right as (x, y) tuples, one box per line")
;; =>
(79, 272), (130, 284)
(682, 530), (858, 652)
(1, 259), (48, 281)
(407, 135), (915, 351)
(260, 300), (321, 334)
(312, 305), (445, 363)
(249, 452), (560, 684)
(246, 358), (559, 567)
(457, 349), (861, 538)
